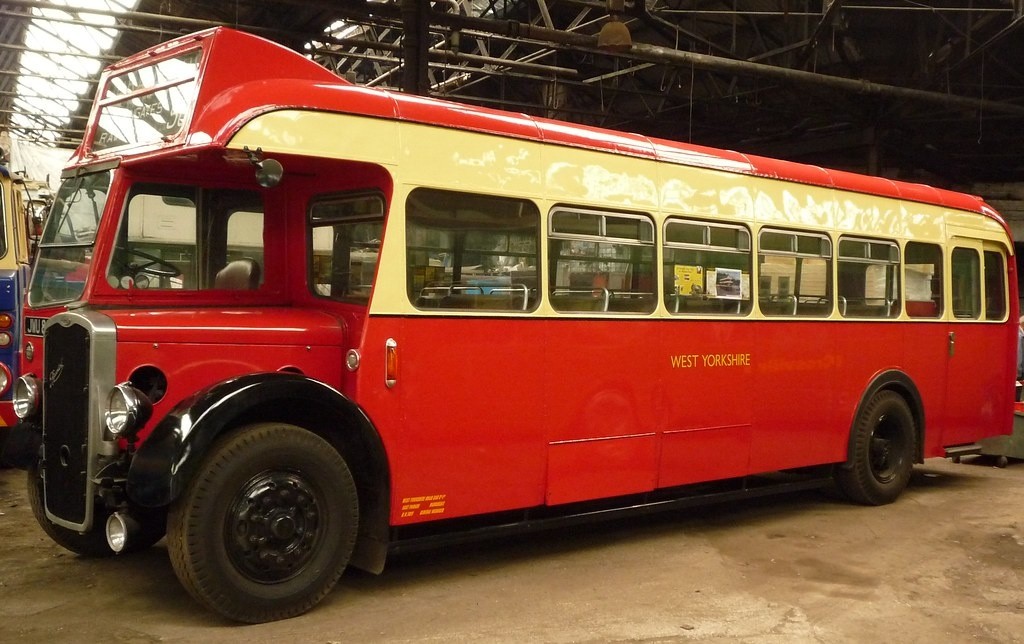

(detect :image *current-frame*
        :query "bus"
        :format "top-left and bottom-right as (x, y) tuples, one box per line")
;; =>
(11, 25), (1020, 625)
(0, 147), (32, 428)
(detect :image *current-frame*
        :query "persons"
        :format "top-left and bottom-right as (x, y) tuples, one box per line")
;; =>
(1016, 315), (1024, 402)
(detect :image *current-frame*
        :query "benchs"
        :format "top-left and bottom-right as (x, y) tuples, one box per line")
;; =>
(412, 279), (944, 314)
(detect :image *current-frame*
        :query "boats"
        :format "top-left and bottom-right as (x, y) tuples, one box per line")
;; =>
(721, 278), (734, 291)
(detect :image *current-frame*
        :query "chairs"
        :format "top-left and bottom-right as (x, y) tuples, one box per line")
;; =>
(213, 257), (261, 290)
